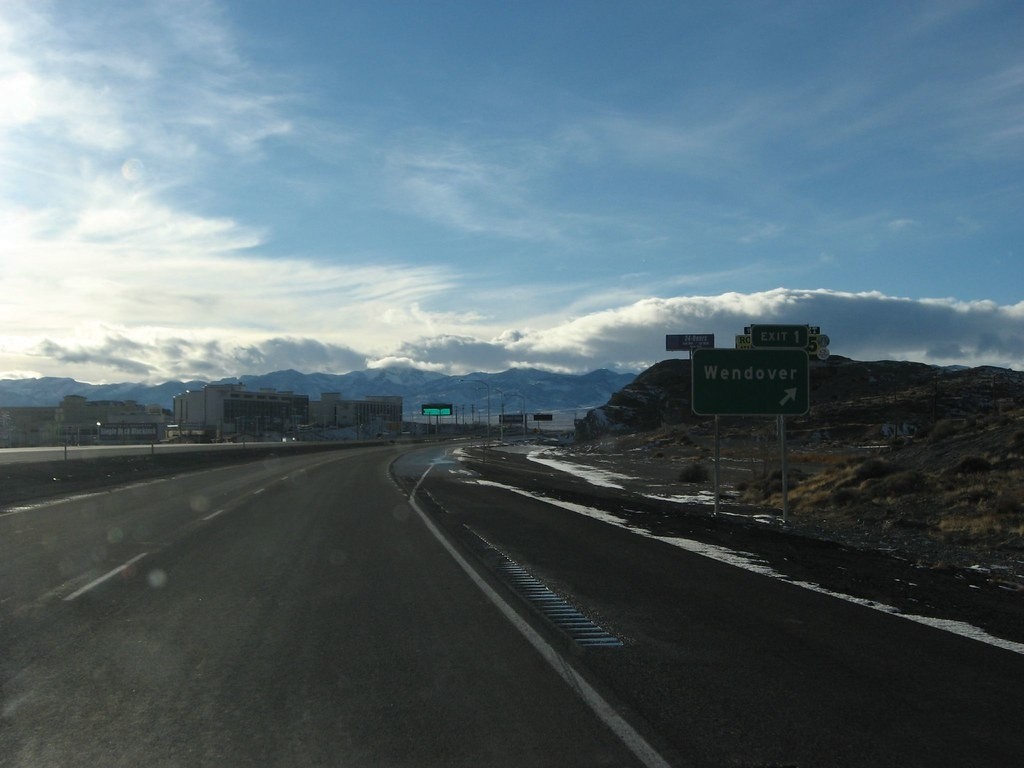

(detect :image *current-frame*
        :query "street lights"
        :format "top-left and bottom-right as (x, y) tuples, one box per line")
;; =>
(508, 394), (525, 440)
(476, 387), (503, 444)
(459, 379), (490, 448)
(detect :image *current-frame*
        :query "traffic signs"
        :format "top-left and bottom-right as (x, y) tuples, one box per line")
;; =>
(692, 348), (810, 417)
